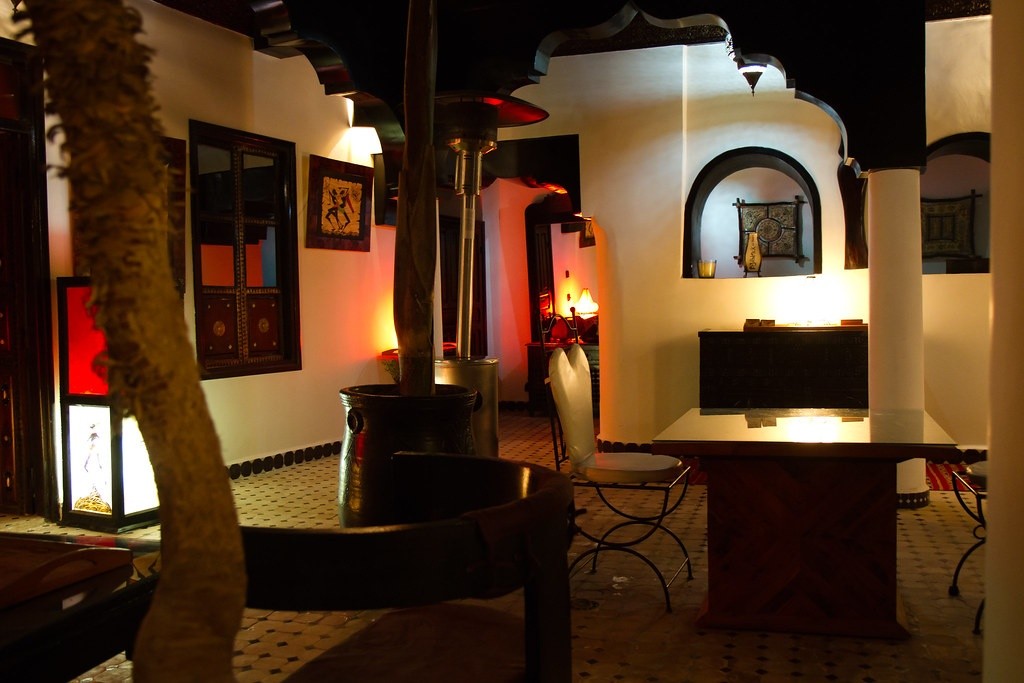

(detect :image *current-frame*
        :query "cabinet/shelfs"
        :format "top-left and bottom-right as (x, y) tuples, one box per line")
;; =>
(56, 275), (160, 535)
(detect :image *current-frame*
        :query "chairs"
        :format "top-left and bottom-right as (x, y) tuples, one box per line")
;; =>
(949, 458), (989, 633)
(130, 448), (574, 683)
(538, 307), (694, 612)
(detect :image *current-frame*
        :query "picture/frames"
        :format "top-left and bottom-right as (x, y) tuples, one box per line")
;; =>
(304, 152), (373, 252)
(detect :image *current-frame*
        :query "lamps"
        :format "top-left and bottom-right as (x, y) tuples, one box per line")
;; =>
(574, 288), (599, 314)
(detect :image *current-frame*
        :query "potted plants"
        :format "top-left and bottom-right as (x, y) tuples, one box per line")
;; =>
(337, 0), (478, 531)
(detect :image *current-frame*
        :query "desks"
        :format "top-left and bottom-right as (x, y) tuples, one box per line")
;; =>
(0, 540), (157, 682)
(655, 407), (957, 640)
